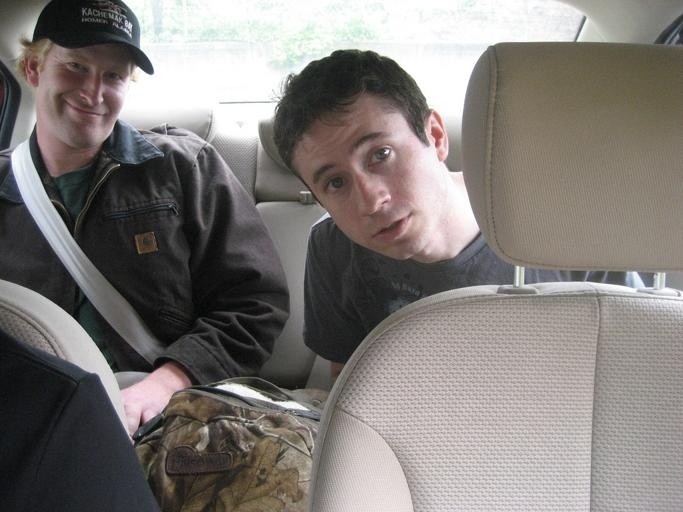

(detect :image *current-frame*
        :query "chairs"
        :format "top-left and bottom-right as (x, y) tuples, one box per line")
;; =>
(0, 276), (133, 458)
(117, 101), (458, 392)
(303, 41), (681, 510)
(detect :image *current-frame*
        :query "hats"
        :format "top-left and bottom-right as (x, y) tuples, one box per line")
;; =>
(32, 1), (153, 75)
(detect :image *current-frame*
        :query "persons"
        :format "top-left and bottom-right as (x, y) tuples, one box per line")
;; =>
(271, 48), (659, 395)
(0, 332), (169, 512)
(1, 1), (291, 448)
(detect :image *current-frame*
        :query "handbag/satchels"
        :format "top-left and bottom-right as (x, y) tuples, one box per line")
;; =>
(131, 375), (325, 511)
